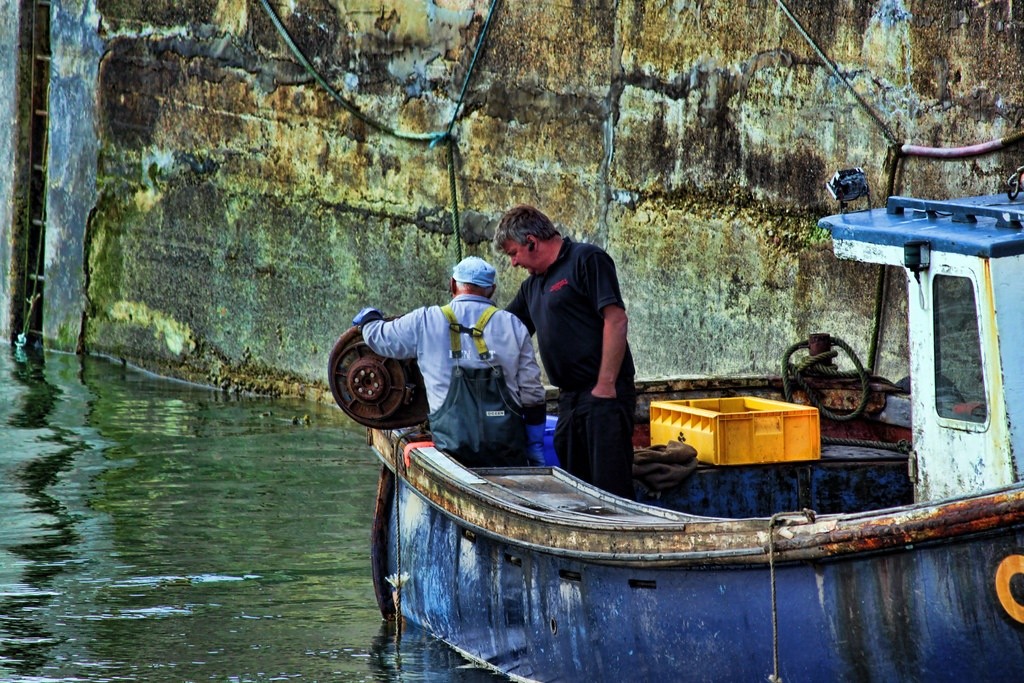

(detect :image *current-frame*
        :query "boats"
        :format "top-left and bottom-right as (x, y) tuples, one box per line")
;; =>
(325, 161), (1024, 683)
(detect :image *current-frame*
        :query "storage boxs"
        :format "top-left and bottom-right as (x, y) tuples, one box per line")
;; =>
(647, 396), (822, 466)
(545, 415), (562, 468)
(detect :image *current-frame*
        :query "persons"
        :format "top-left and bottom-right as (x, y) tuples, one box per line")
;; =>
(354, 256), (547, 466)
(494, 205), (638, 500)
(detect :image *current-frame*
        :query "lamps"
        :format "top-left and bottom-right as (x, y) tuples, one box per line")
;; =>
(826, 167), (874, 213)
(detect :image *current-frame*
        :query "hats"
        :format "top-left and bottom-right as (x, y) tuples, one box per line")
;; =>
(452, 255), (496, 288)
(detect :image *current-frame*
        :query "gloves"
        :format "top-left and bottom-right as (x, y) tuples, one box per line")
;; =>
(351, 307), (384, 326)
(521, 422), (546, 467)
